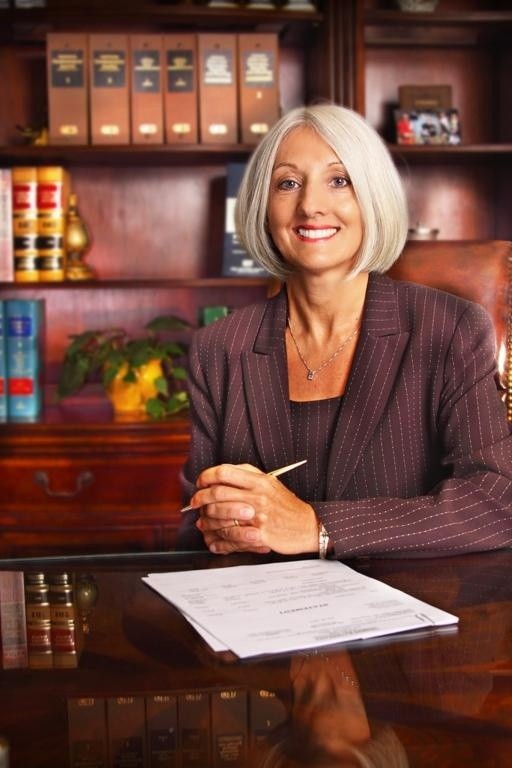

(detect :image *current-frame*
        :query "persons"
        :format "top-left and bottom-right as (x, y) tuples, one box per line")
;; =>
(184, 103), (512, 559)
(393, 108), (462, 147)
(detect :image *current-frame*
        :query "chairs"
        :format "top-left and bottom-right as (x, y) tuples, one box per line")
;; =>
(383, 239), (512, 426)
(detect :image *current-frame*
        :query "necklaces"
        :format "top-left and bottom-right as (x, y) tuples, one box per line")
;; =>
(287, 318), (360, 381)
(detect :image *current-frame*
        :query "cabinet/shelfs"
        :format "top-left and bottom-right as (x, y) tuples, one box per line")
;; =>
(0, 0), (512, 559)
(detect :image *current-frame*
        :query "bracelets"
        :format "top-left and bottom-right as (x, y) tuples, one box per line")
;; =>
(318, 521), (330, 559)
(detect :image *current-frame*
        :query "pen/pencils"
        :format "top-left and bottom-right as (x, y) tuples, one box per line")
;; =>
(179, 460), (308, 513)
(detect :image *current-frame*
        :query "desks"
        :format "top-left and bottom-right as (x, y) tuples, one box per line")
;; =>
(0, 550), (512, 768)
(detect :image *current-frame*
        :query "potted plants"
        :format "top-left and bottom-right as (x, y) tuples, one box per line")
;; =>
(53, 315), (192, 425)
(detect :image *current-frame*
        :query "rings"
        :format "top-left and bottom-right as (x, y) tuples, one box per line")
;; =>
(234, 519), (240, 526)
